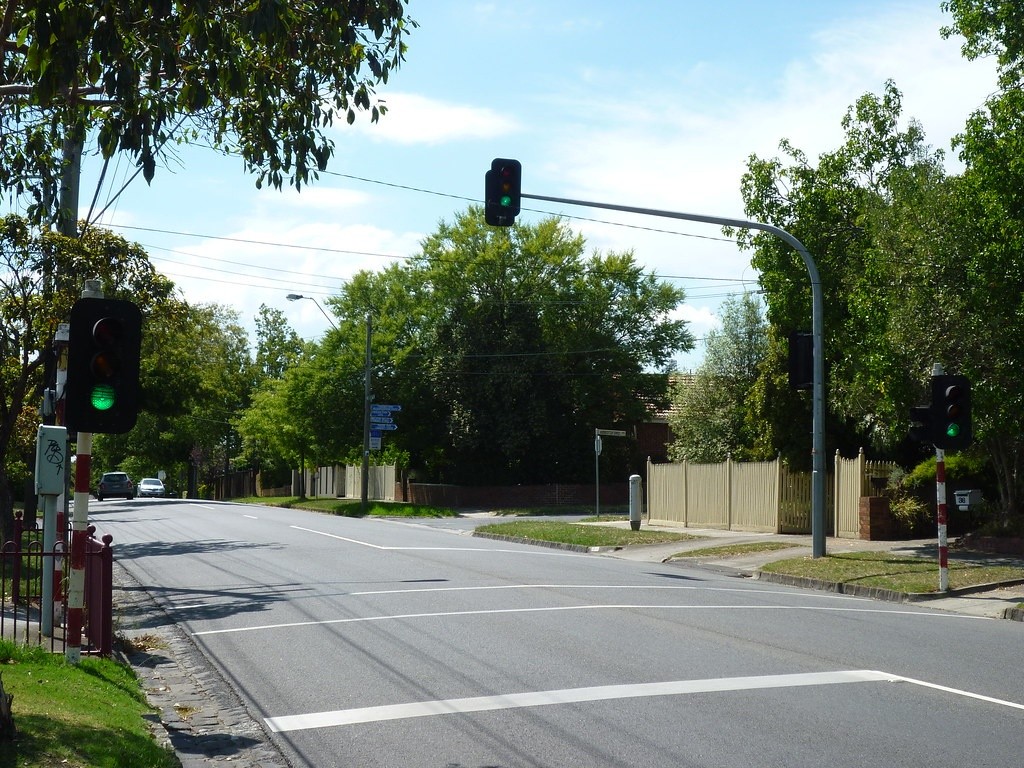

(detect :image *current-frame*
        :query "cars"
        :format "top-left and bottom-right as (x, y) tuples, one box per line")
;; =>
(136, 477), (167, 498)
(95, 471), (134, 501)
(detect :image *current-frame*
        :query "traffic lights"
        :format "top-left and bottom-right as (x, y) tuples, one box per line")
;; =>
(63, 297), (152, 435)
(492, 158), (522, 216)
(931, 375), (973, 452)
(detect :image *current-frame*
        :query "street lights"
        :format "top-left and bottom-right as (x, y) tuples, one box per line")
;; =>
(286, 294), (372, 513)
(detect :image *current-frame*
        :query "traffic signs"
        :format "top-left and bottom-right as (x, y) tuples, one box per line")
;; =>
(369, 405), (401, 450)
